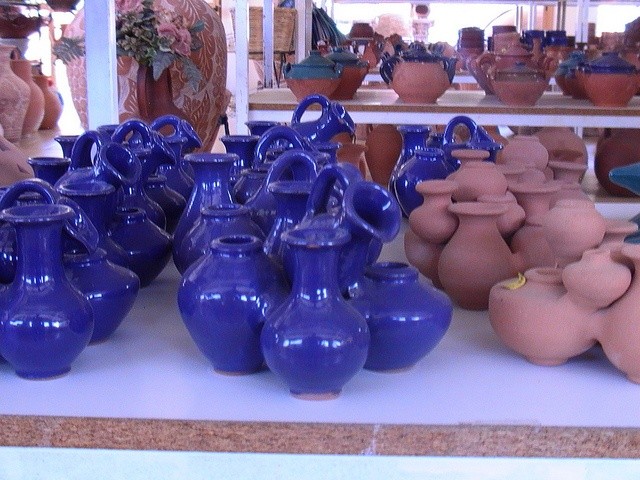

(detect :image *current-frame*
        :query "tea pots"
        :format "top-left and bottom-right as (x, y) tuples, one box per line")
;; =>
(552, 43), (639, 109)
(377, 40), (459, 102)
(465, 37), (559, 108)
(279, 43), (369, 109)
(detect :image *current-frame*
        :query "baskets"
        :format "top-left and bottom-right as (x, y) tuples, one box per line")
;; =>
(231, 6), (297, 53)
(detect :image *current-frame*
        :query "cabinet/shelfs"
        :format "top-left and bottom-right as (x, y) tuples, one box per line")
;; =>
(234, 1), (639, 223)
